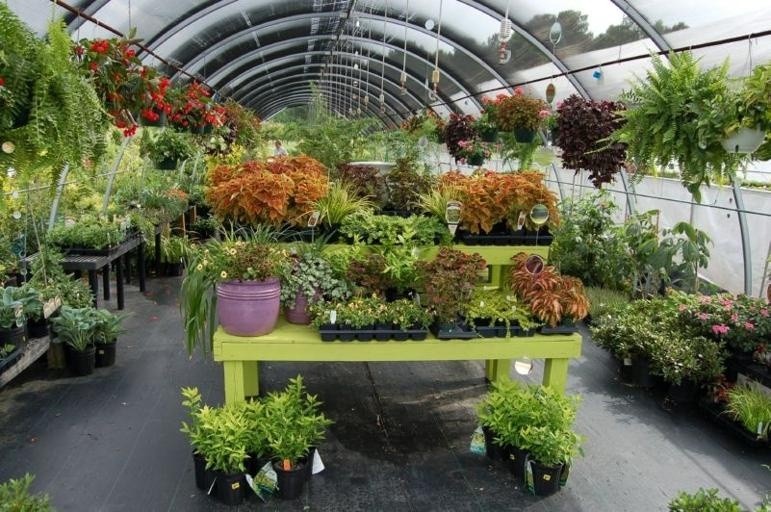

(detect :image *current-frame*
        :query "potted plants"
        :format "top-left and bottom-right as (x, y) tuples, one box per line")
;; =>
(142, 233), (199, 277)
(585, 39), (771, 205)
(45, 214), (131, 257)
(0, 270), (134, 377)
(588, 298), (771, 450)
(473, 371), (585, 498)
(177, 151), (591, 344)
(0, 0), (116, 200)
(175, 372), (338, 505)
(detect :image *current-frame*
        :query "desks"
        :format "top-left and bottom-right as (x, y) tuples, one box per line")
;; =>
(17, 224), (164, 310)
(159, 201), (197, 264)
(212, 314), (583, 407)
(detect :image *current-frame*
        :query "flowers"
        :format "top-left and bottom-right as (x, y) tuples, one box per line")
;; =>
(465, 85), (564, 132)
(457, 131), (501, 165)
(677, 290), (771, 353)
(69, 26), (230, 137)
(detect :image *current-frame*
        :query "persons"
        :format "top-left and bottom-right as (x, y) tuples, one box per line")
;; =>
(274, 139), (286, 155)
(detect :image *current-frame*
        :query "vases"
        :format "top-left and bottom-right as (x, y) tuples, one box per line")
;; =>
(514, 127), (535, 142)
(479, 128), (498, 142)
